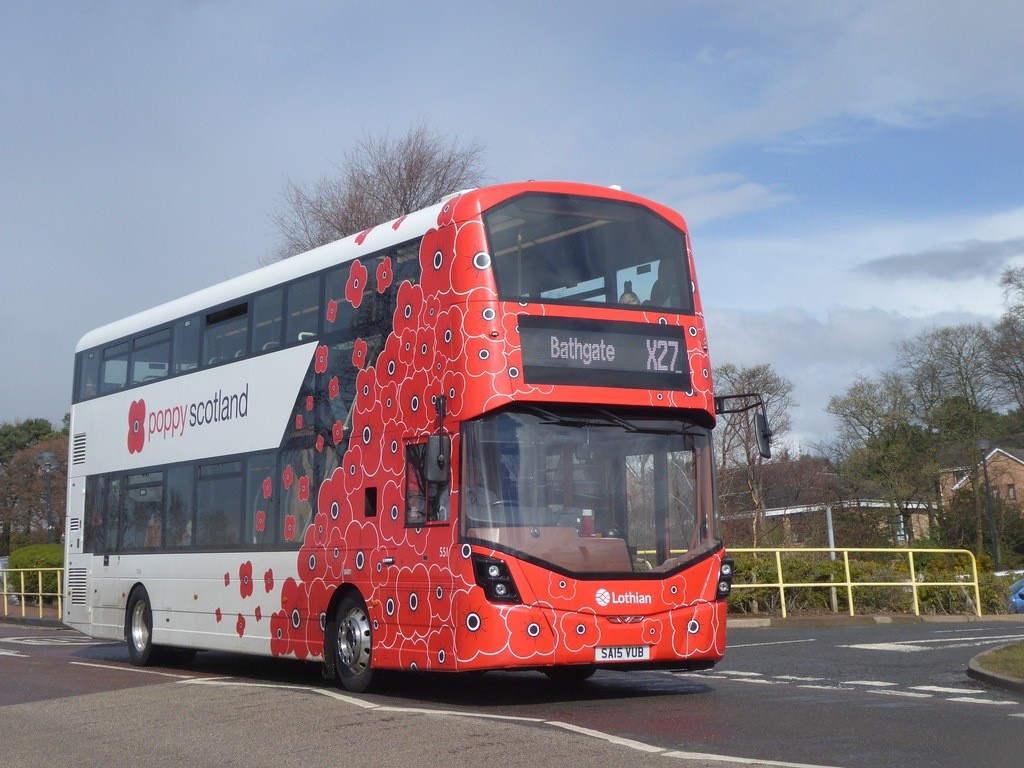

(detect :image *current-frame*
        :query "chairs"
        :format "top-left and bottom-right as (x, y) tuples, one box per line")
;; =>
(140, 331), (318, 388)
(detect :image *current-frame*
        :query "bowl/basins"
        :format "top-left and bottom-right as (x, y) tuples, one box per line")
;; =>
(602, 528), (619, 538)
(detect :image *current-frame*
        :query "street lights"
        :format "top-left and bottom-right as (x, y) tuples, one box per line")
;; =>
(976, 439), (1001, 573)
(36, 450), (56, 546)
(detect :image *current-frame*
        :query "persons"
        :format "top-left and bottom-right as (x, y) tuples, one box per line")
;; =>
(438, 456), (498, 521)
(182, 520), (191, 545)
(619, 291), (642, 305)
(144, 511), (161, 546)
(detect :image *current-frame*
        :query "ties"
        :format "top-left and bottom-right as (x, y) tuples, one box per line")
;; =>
(469, 491), (478, 518)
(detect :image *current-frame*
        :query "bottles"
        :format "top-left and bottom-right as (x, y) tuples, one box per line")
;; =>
(581, 509), (593, 538)
(619, 280), (641, 306)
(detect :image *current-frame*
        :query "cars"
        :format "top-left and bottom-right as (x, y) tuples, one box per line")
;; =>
(1003, 576), (1024, 614)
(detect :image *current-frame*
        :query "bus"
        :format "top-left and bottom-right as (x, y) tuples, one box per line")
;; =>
(61, 179), (776, 694)
